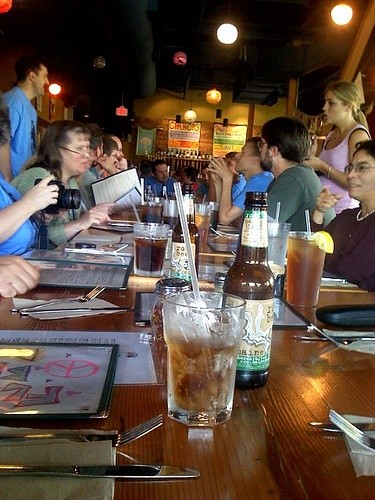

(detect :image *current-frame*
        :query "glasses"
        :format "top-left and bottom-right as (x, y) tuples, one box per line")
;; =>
(342, 163), (375, 176)
(256, 140), (268, 148)
(106, 153), (119, 160)
(55, 144), (96, 157)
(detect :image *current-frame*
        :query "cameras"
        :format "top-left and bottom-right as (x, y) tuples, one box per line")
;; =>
(34, 178), (82, 215)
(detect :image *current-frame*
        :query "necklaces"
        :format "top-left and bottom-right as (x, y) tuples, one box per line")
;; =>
(356, 209), (374, 222)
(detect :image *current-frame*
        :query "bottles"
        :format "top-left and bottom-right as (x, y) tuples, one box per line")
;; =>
(135, 147), (220, 177)
(171, 184), (199, 288)
(149, 276), (193, 344)
(222, 190), (275, 390)
(213, 272), (226, 293)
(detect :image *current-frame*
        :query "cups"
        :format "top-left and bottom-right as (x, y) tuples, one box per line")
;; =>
(142, 202), (163, 225)
(133, 221), (170, 277)
(267, 222), (291, 282)
(163, 291), (247, 428)
(286, 231), (326, 309)
(208, 201), (221, 232)
(163, 199), (179, 226)
(194, 203), (213, 254)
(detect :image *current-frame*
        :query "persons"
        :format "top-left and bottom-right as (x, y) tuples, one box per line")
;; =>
(259, 116), (337, 231)
(298, 81), (372, 220)
(207, 136), (275, 227)
(73, 125), (130, 215)
(137, 151), (241, 201)
(0, 53), (118, 299)
(308, 139), (375, 294)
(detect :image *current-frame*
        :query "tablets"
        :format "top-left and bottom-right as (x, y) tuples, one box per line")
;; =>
(222, 259), (345, 285)
(207, 241), (238, 253)
(134, 291), (155, 327)
(271, 295), (311, 330)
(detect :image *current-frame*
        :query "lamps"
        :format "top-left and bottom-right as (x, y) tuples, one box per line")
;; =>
(116, 92), (128, 116)
(93, 55), (105, 68)
(173, 51), (188, 66)
(206, 84), (221, 105)
(184, 93), (197, 123)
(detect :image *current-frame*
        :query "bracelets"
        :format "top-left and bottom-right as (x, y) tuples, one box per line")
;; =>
(327, 164), (332, 180)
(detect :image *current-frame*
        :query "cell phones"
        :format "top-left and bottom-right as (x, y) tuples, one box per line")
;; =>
(76, 242), (97, 250)
(100, 242), (129, 253)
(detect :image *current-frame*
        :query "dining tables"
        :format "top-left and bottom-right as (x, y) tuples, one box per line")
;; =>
(0, 193), (375, 500)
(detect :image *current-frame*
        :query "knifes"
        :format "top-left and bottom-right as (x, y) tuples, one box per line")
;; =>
(308, 421), (374, 432)
(19, 306), (136, 315)
(289, 334), (375, 343)
(0, 464), (201, 479)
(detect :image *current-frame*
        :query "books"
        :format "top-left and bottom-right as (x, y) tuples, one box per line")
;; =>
(1, 249), (311, 419)
(91, 165), (146, 209)
(208, 242), (241, 253)
(198, 260), (234, 280)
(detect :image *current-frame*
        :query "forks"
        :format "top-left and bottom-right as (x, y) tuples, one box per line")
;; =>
(310, 322), (375, 348)
(110, 219), (144, 227)
(0, 414), (164, 447)
(63, 243), (129, 255)
(11, 285), (106, 312)
(328, 408), (375, 453)
